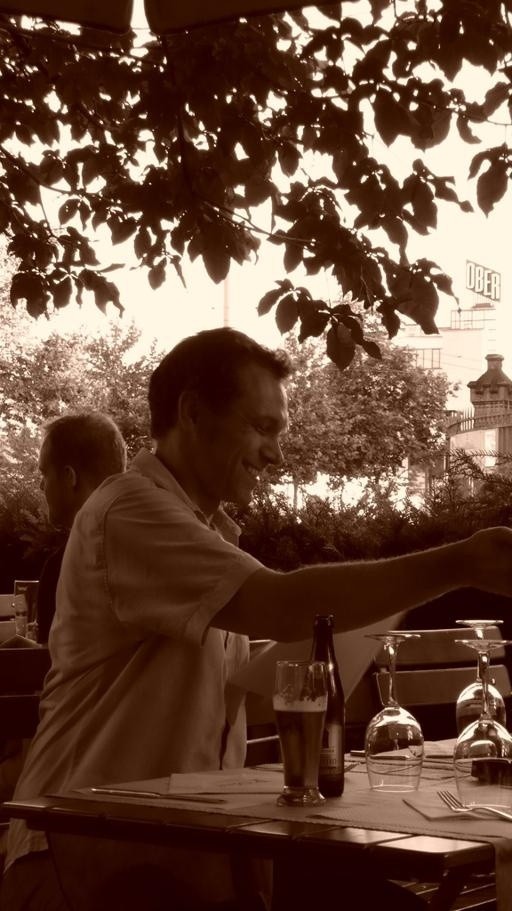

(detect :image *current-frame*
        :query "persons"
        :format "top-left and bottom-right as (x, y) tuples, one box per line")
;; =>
(0, 327), (511, 911)
(29, 409), (129, 647)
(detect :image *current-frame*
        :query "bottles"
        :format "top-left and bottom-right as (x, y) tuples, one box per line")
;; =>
(302, 612), (347, 798)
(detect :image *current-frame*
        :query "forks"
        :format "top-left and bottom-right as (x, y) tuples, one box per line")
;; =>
(251, 763), (356, 774)
(436, 790), (512, 823)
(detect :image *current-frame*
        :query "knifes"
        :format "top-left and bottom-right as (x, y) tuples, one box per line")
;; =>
(350, 748), (454, 764)
(90, 785), (226, 805)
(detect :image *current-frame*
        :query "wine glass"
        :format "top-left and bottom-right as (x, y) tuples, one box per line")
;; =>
(456, 635), (512, 813)
(456, 618), (507, 739)
(362, 632), (426, 792)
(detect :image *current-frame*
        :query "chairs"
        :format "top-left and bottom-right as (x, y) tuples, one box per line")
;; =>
(374, 626), (512, 750)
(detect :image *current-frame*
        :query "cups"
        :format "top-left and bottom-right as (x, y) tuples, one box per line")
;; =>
(13, 579), (40, 644)
(272, 659), (328, 808)
